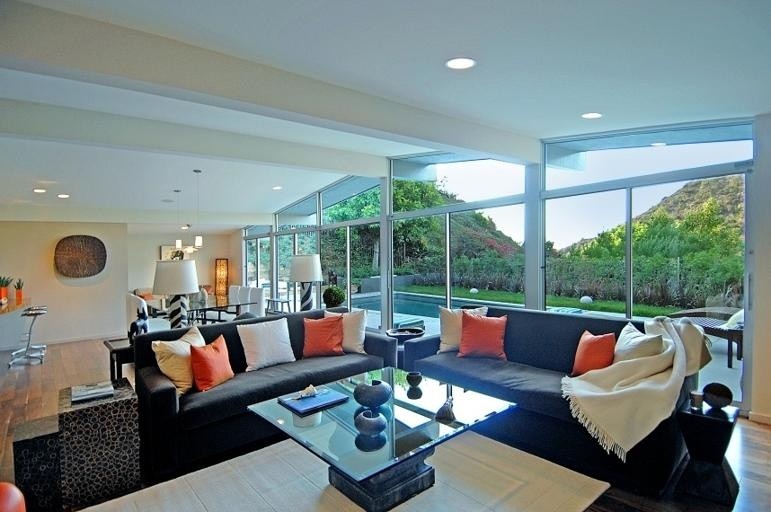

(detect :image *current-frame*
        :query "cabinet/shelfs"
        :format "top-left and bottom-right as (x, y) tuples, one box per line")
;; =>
(58, 378), (140, 512)
(13, 415), (62, 512)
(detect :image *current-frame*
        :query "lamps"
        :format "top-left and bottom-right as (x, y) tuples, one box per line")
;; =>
(174, 190), (182, 249)
(152, 260), (199, 330)
(194, 170), (202, 248)
(286, 254), (323, 311)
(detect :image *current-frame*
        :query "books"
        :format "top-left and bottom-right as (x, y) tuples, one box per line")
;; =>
(70, 379), (115, 401)
(276, 382), (352, 418)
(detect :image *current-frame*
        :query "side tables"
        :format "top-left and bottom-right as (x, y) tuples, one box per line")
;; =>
(674, 401), (739, 512)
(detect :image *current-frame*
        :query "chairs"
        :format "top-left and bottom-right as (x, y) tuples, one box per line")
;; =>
(127, 286), (265, 337)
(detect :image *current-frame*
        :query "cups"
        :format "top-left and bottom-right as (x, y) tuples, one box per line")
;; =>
(690, 389), (705, 412)
(407, 372), (422, 386)
(407, 385), (423, 399)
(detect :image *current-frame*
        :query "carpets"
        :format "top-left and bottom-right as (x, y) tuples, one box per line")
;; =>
(76, 403), (611, 512)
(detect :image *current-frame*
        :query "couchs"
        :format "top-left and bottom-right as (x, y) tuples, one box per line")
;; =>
(133, 305), (398, 488)
(404, 305), (697, 502)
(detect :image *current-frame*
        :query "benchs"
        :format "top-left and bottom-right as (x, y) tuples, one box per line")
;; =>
(266, 298), (291, 315)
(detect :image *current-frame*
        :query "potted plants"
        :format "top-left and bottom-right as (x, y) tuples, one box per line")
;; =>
(0, 276), (25, 305)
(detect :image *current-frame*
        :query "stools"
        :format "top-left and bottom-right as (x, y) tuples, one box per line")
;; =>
(8, 306), (47, 368)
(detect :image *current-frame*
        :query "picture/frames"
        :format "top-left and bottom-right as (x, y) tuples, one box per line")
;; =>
(215, 258), (229, 295)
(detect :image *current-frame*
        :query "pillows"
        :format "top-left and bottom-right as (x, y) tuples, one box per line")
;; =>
(437, 306), (662, 376)
(151, 309), (368, 412)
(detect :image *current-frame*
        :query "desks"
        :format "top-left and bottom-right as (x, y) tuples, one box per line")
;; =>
(104, 337), (135, 382)
(398, 341), (403, 369)
